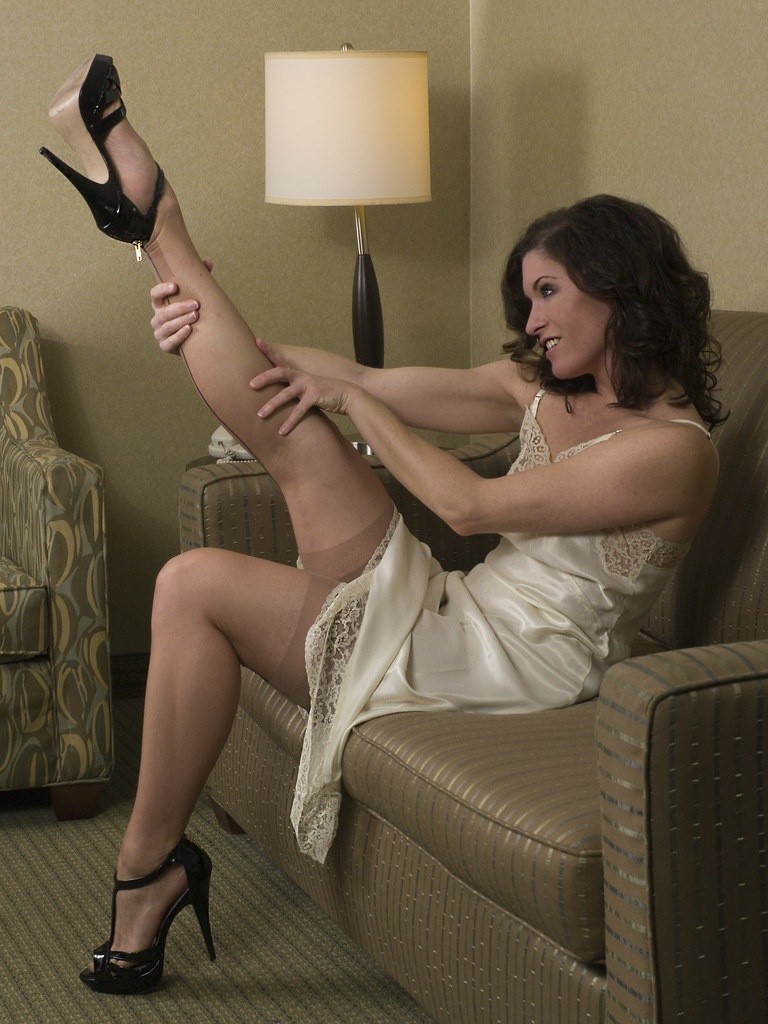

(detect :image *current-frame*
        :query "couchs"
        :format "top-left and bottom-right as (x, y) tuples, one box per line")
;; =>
(0, 305), (118, 822)
(177, 308), (768, 1024)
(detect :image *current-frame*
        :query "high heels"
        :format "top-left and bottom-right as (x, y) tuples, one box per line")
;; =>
(38, 53), (164, 261)
(79, 833), (216, 994)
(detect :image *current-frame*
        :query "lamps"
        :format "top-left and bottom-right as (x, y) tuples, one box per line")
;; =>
(266, 42), (432, 456)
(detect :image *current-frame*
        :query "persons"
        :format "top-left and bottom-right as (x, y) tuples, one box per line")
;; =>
(39, 55), (730, 995)
